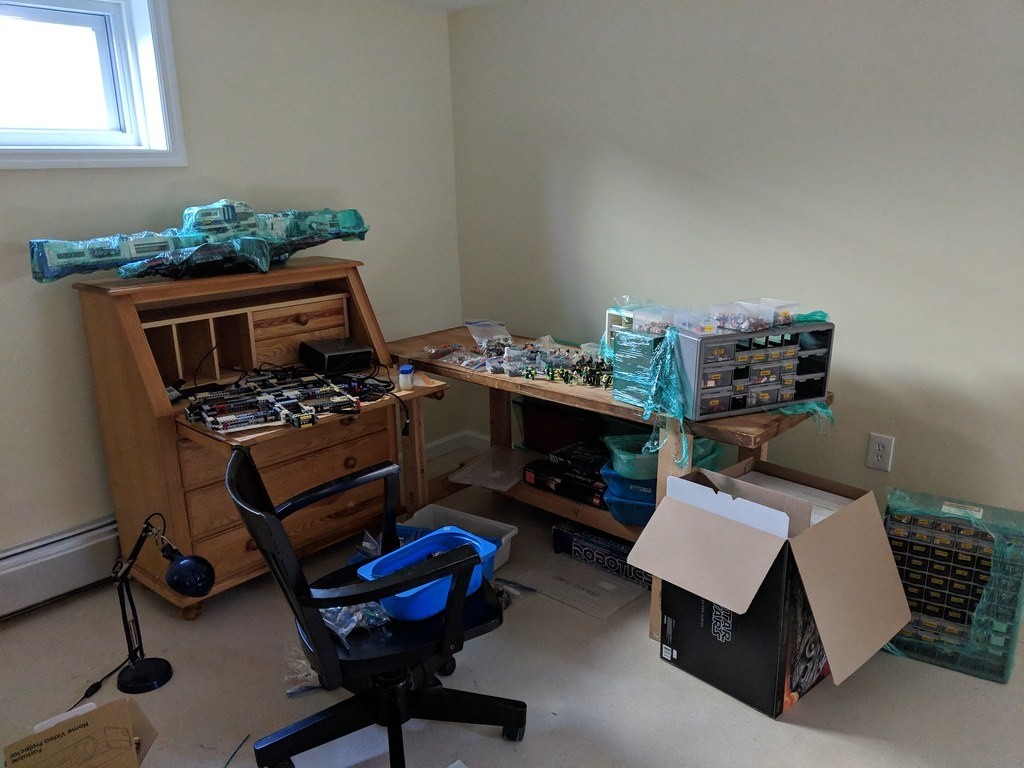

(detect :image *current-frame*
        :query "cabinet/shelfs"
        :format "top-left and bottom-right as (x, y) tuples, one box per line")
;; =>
(72, 256), (452, 622)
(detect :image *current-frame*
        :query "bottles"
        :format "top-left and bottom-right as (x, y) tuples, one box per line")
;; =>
(398, 365), (414, 391)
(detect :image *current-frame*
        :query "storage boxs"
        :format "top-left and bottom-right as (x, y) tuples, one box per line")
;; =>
(551, 519), (652, 592)
(625, 456), (912, 719)
(356, 525), (497, 622)
(523, 457), (609, 511)
(396, 503), (519, 571)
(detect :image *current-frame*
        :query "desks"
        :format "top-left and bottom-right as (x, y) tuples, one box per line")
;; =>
(385, 322), (833, 516)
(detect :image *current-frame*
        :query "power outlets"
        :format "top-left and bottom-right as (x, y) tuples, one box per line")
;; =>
(865, 431), (895, 472)
